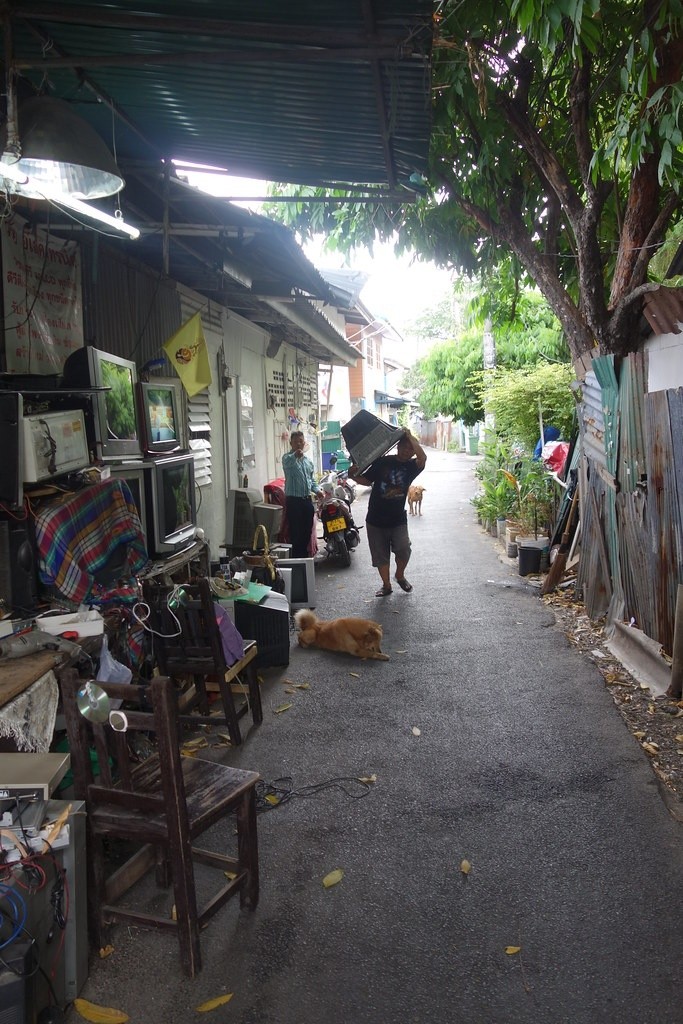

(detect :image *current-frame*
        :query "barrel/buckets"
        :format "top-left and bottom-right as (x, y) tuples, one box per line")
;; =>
(322, 452), (336, 471)
(517, 547), (542, 576)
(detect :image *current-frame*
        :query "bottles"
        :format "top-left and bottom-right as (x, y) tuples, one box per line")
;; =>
(219, 557), (231, 581)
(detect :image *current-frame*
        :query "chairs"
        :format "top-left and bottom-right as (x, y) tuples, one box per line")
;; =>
(59, 668), (260, 979)
(144, 578), (263, 745)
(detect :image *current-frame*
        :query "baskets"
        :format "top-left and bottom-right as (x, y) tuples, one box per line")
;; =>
(242, 523), (279, 565)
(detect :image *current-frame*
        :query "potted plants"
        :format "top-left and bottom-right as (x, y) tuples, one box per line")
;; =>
(468, 420), (556, 573)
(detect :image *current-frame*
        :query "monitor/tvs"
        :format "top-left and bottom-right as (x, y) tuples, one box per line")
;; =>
(213, 583), (290, 668)
(225, 488), (283, 549)
(341, 410), (407, 477)
(63, 347), (197, 560)
(273, 557), (318, 610)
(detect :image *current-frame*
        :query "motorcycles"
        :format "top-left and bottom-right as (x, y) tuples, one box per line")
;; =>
(319, 457), (365, 568)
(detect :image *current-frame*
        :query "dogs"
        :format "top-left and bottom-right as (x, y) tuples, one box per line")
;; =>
(407, 486), (427, 517)
(294, 609), (391, 660)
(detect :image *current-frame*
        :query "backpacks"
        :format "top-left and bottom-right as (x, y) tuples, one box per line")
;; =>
(213, 603), (244, 667)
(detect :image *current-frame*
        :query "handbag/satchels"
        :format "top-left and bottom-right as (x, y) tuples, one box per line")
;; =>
(96, 634), (132, 711)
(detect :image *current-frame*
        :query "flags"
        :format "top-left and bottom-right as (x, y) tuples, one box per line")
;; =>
(163, 311), (213, 399)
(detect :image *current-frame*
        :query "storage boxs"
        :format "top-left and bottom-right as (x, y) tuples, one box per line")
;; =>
(35, 609), (105, 636)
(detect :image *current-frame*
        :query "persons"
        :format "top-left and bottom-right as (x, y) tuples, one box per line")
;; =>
(347, 426), (427, 597)
(282, 431), (326, 558)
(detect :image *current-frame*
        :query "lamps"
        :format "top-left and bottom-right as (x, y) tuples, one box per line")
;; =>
(0, 164), (141, 241)
(0, 65), (126, 200)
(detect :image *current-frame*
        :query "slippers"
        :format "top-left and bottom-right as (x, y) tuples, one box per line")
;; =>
(396, 575), (412, 593)
(376, 587), (392, 596)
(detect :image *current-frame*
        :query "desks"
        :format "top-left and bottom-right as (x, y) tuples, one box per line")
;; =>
(146, 536), (211, 586)
(0, 799), (90, 1015)
(0, 637), (105, 709)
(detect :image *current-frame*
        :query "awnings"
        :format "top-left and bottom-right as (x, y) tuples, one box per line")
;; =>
(384, 357), (411, 374)
(375, 391), (411, 407)
(0, 0), (439, 199)
(374, 315), (403, 344)
(118, 166), (379, 368)
